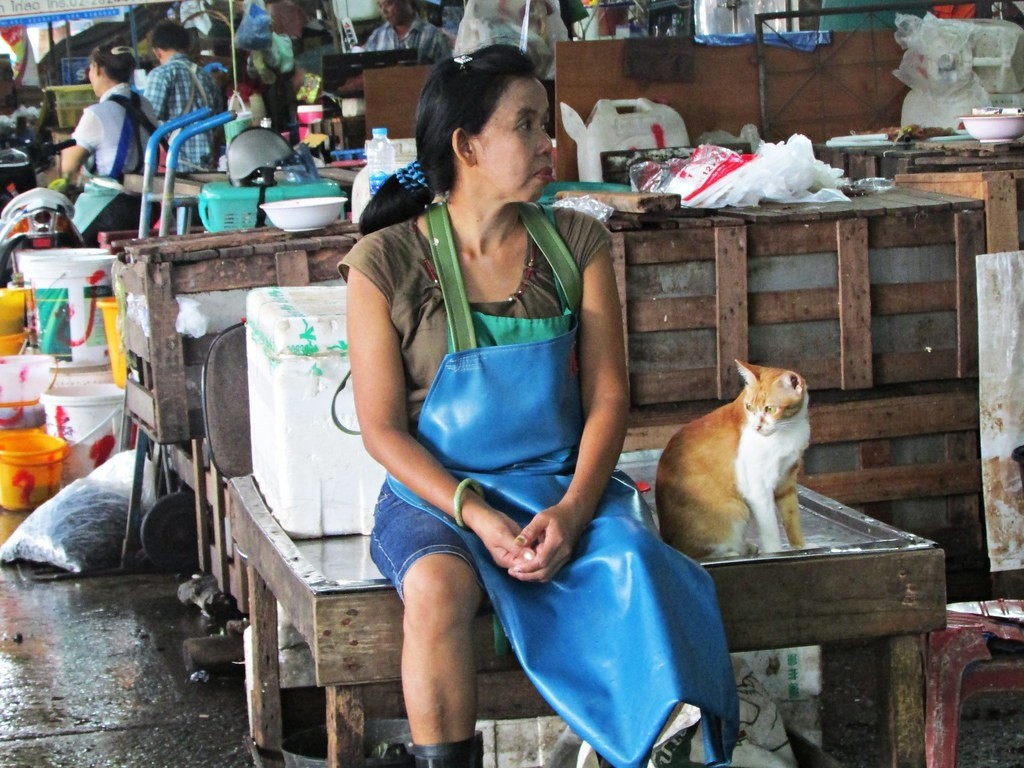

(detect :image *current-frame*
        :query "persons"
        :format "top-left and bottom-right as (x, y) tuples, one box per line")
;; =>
(338, 44), (740, 768)
(357, 0), (452, 62)
(144, 20), (222, 175)
(54, 39), (169, 248)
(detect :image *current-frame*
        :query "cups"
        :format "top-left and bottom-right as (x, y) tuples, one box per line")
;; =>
(297, 104), (323, 142)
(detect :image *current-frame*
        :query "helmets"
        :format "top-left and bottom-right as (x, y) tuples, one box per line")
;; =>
(228, 127), (294, 186)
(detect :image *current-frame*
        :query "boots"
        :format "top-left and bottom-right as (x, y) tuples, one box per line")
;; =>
(411, 732), (484, 768)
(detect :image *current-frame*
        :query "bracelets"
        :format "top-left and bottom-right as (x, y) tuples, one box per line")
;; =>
(453, 476), (484, 528)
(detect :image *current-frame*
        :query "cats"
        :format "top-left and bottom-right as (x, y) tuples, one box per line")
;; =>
(655, 357), (812, 565)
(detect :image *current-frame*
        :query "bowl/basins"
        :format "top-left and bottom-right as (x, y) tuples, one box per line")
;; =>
(958, 115), (1024, 144)
(258, 197), (349, 232)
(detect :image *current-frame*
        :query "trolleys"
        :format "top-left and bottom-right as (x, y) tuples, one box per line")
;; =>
(14, 108), (241, 584)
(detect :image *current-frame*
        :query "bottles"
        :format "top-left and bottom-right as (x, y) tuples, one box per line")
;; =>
(576, 94), (692, 184)
(367, 128), (397, 196)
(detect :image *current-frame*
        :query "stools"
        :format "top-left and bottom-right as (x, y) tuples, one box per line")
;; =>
(921, 599), (1024, 767)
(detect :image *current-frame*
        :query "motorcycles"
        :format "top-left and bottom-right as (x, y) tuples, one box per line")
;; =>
(0, 137), (77, 287)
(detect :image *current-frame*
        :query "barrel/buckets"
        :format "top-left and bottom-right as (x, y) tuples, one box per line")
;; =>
(0, 248), (133, 548)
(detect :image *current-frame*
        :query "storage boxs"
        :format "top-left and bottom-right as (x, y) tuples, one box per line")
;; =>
(48, 85), (98, 129)
